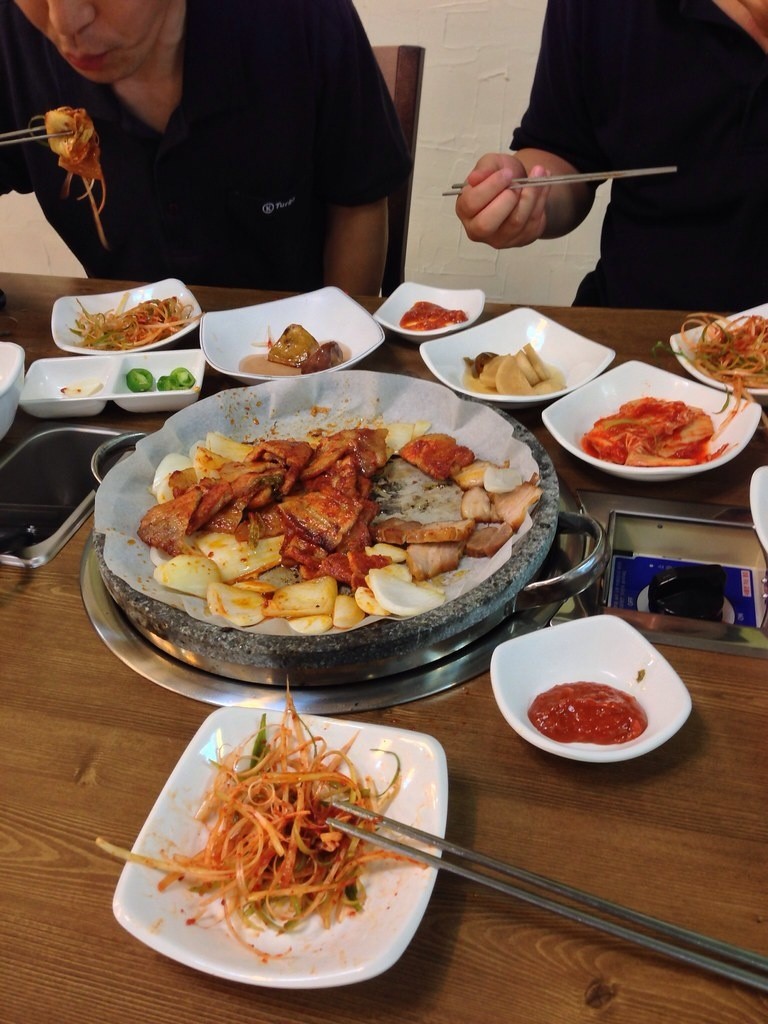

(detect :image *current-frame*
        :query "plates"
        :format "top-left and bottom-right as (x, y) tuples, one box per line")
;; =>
(373, 282), (485, 343)
(419, 307), (616, 410)
(749, 466), (768, 552)
(490, 615), (692, 764)
(670, 303), (768, 407)
(112, 705), (448, 989)
(19, 349), (206, 418)
(542, 360), (762, 481)
(51, 278), (203, 355)
(199, 287), (385, 385)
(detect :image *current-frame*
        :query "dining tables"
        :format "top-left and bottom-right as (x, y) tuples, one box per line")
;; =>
(0, 270), (768, 1024)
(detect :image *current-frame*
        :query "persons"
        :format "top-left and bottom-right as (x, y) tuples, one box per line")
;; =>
(455, 0), (768, 310)
(0, 0), (414, 298)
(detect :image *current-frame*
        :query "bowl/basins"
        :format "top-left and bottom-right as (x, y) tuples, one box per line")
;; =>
(0, 341), (25, 440)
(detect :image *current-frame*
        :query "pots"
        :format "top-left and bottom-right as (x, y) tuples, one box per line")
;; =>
(91, 396), (609, 689)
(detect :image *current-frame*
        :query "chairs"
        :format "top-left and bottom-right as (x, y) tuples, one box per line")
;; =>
(371, 44), (425, 298)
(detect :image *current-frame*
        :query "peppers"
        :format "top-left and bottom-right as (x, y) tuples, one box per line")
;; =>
(170, 368), (195, 389)
(157, 376), (179, 392)
(126, 367), (153, 392)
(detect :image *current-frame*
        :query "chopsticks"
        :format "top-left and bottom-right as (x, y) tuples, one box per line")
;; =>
(0, 126), (73, 146)
(326, 798), (768, 994)
(441, 166), (677, 196)
